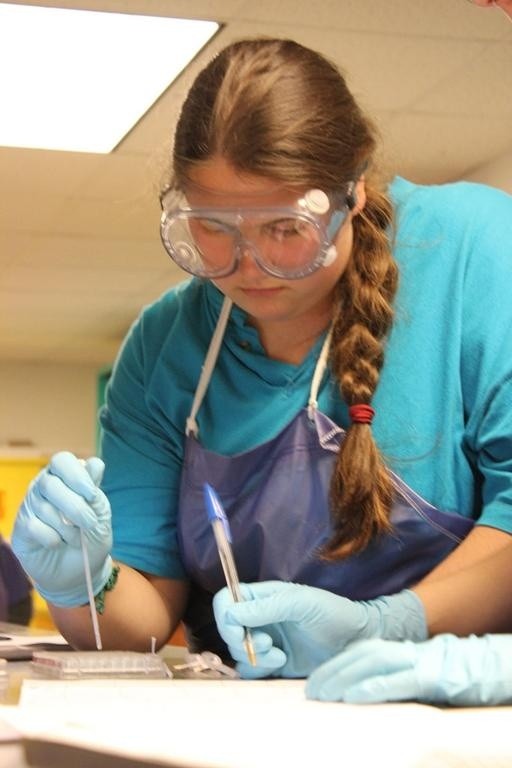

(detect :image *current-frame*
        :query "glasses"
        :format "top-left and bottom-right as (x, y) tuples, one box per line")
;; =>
(155, 181), (353, 279)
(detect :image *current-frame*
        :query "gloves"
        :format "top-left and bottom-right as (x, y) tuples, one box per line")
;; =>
(306, 634), (511, 705)
(213, 581), (427, 679)
(12, 452), (115, 608)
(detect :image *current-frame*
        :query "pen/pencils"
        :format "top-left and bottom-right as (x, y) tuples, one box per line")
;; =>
(204, 482), (256, 665)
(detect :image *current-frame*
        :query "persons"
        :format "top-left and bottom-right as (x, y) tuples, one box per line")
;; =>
(212, 538), (512, 708)
(10, 38), (512, 652)
